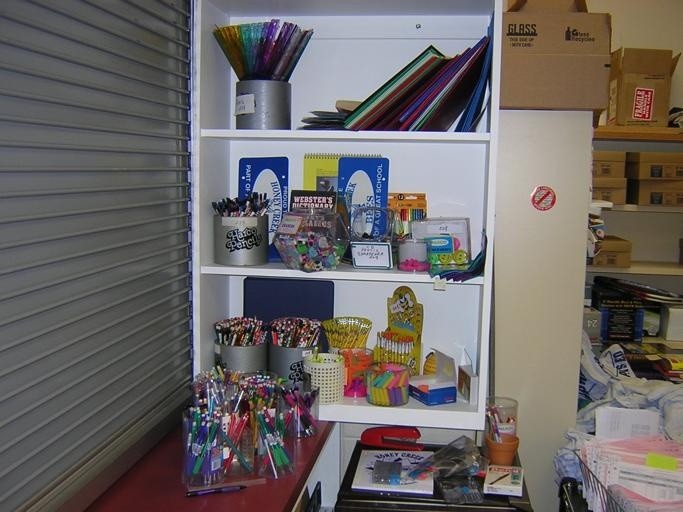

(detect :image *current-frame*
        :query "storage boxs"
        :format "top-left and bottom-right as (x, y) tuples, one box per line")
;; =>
(587, 42), (682, 352)
(498, 10), (614, 112)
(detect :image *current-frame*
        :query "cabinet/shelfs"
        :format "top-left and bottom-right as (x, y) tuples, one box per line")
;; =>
(188, 0), (507, 433)
(304, 421), (486, 511)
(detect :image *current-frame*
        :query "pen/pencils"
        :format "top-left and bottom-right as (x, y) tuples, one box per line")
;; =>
(377, 331), (414, 365)
(322, 317), (372, 349)
(400, 209), (423, 222)
(485, 405), (517, 443)
(269, 317), (323, 348)
(185, 485), (248, 497)
(489, 467), (521, 473)
(212, 192), (273, 217)
(214, 317), (269, 346)
(183, 360), (320, 488)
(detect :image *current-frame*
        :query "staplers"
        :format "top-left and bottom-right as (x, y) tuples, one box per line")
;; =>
(361, 426), (424, 450)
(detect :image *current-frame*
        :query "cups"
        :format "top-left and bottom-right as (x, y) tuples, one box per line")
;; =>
(337, 348), (409, 405)
(236, 80), (291, 129)
(484, 396), (519, 464)
(213, 215), (268, 267)
(181, 336), (321, 487)
(397, 241), (431, 271)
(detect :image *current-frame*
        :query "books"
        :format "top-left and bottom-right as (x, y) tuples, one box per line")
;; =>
(294, 37), (489, 132)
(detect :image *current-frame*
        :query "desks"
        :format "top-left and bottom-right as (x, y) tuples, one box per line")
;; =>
(331, 436), (535, 512)
(73, 417), (341, 511)
